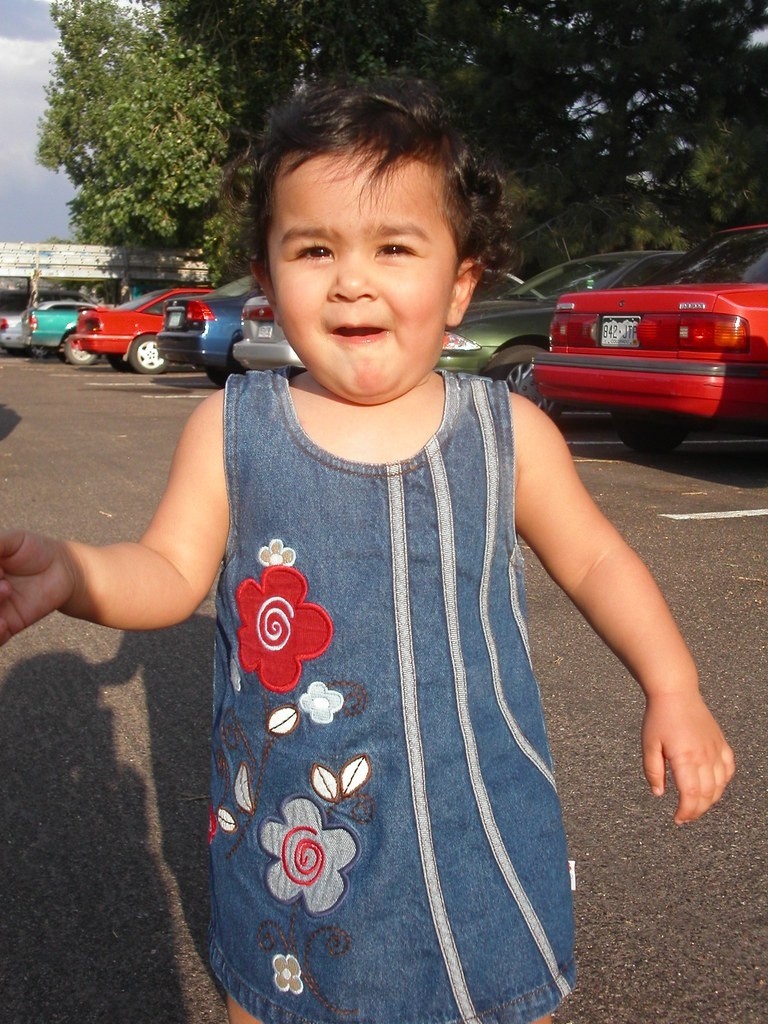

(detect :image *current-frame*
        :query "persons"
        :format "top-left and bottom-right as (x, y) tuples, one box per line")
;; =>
(0, 81), (734, 1024)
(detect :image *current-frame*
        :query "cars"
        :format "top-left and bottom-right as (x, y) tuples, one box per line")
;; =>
(431, 248), (689, 425)
(71, 288), (215, 375)
(0, 300), (100, 358)
(532, 225), (768, 454)
(156, 276), (266, 388)
(234, 264), (550, 373)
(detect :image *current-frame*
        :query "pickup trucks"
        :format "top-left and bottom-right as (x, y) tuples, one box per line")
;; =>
(23, 310), (98, 367)
